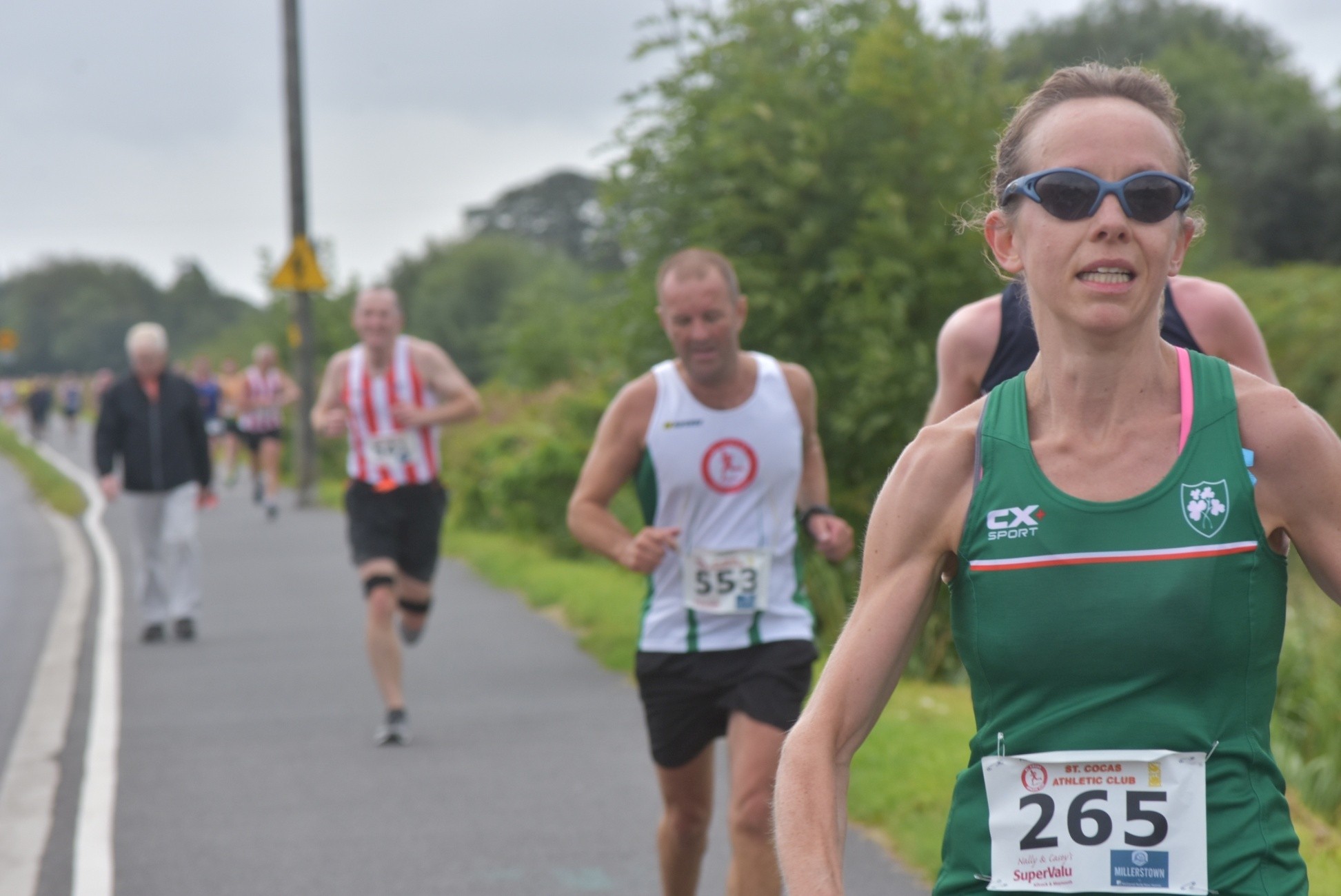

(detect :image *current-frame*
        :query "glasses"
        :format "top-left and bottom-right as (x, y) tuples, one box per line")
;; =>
(1000, 167), (1194, 223)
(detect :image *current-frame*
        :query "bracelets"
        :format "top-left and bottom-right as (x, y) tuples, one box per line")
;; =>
(799, 506), (833, 529)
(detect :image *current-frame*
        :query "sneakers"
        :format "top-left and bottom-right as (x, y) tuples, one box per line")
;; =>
(377, 723), (405, 745)
(399, 610), (424, 644)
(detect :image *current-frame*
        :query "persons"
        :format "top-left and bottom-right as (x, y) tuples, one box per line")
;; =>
(564, 245), (854, 896)
(0, 345), (301, 518)
(307, 288), (484, 744)
(912, 273), (1289, 427)
(94, 321), (214, 644)
(771, 63), (1341, 896)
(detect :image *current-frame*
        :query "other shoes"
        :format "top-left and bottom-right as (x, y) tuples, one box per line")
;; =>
(143, 626), (164, 642)
(175, 619), (195, 639)
(255, 482), (262, 501)
(266, 506), (277, 519)
